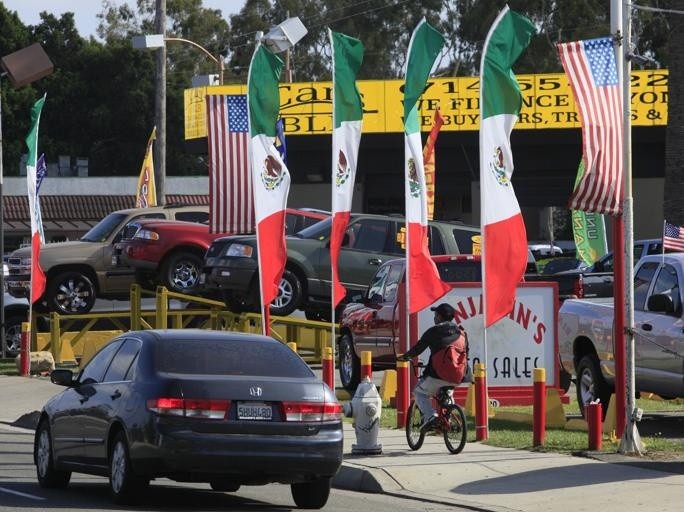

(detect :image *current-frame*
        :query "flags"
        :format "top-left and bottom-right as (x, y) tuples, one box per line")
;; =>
(662, 222), (684, 252)
(556, 36), (625, 217)
(204, 94), (256, 234)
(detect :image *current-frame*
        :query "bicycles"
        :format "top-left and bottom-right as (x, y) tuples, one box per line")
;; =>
(400, 356), (468, 455)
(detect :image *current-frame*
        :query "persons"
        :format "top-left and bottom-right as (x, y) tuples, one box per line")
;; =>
(397, 303), (469, 434)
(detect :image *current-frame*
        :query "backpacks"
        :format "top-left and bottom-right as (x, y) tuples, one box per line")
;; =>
(430, 323), (468, 385)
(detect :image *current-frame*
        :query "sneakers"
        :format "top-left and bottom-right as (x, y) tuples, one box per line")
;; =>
(418, 412), (440, 435)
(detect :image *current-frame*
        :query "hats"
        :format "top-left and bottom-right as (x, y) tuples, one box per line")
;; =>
(430, 303), (455, 318)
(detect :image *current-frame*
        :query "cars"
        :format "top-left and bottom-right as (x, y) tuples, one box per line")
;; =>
(0, 264), (61, 357)
(31, 327), (345, 509)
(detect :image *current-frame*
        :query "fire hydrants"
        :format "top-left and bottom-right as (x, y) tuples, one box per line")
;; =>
(341, 374), (384, 454)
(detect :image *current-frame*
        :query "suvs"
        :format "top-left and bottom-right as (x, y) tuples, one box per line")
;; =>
(195, 212), (539, 317)
(109, 207), (355, 294)
(4, 203), (211, 315)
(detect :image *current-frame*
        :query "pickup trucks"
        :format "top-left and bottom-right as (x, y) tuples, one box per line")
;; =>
(338, 254), (571, 394)
(558, 252), (684, 421)
(526, 237), (684, 300)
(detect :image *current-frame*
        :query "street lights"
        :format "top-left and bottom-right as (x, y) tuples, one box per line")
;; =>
(0, 41), (54, 358)
(129, 35), (226, 86)
